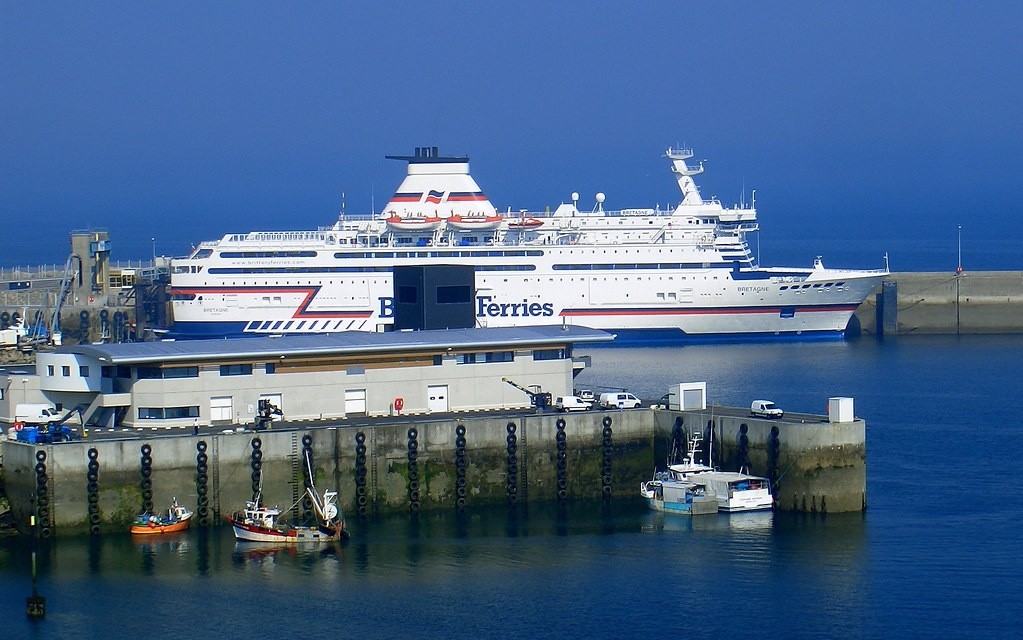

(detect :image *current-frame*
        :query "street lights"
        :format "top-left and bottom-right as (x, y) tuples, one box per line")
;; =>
(958, 224), (963, 267)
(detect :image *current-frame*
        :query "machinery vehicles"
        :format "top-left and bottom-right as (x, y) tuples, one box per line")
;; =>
(501, 377), (553, 408)
(39, 406), (88, 442)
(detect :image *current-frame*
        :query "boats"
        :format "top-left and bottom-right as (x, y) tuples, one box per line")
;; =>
(131, 497), (194, 534)
(226, 492), (345, 543)
(143, 145), (892, 346)
(667, 431), (775, 513)
(231, 540), (340, 571)
(641, 478), (719, 515)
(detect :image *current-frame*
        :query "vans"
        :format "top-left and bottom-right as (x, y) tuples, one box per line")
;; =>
(577, 390), (595, 403)
(15, 404), (63, 425)
(751, 399), (784, 419)
(555, 396), (593, 413)
(599, 392), (641, 410)
